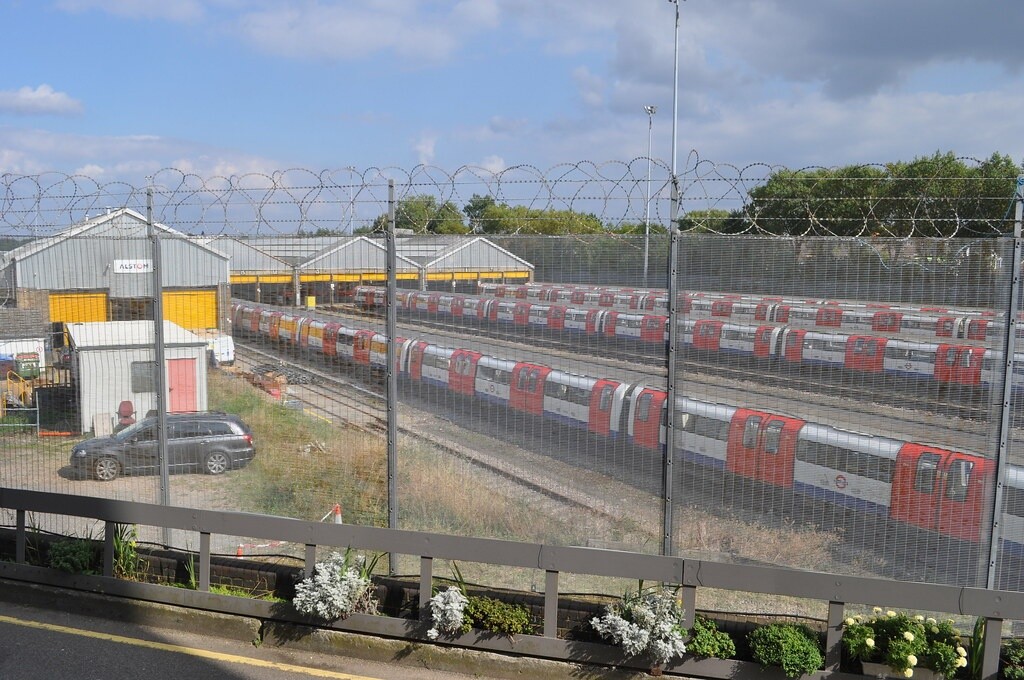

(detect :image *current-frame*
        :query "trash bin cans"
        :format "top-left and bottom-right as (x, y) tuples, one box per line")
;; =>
(15, 352), (40, 380)
(0, 353), (15, 380)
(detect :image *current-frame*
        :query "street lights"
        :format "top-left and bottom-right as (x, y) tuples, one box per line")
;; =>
(643, 103), (659, 287)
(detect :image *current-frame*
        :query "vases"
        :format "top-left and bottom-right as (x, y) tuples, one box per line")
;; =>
(860, 657), (947, 680)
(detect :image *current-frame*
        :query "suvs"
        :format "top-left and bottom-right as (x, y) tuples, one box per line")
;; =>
(69, 409), (256, 480)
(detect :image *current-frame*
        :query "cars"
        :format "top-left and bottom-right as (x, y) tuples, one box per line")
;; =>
(58, 347), (71, 370)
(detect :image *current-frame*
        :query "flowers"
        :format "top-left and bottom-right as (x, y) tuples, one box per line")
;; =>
(426, 585), (470, 640)
(292, 550), (389, 623)
(843, 607), (969, 678)
(588, 586), (688, 666)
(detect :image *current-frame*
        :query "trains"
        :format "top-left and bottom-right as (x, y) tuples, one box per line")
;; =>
(356, 285), (1023, 419)
(479, 282), (1022, 353)
(224, 298), (920, 564)
